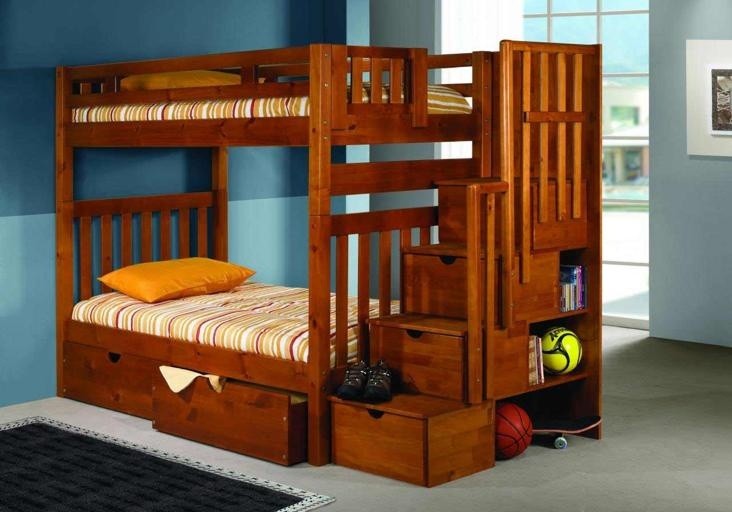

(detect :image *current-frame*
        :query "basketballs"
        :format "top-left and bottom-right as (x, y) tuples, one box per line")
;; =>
(496, 402), (534, 461)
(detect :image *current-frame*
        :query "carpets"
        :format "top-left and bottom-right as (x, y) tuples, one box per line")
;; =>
(0, 416), (335, 512)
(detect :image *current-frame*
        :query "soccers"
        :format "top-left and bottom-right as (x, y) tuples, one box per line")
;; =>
(540, 326), (583, 375)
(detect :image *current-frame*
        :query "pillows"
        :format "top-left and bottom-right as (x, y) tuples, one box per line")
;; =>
(118, 67), (265, 88)
(99, 257), (254, 303)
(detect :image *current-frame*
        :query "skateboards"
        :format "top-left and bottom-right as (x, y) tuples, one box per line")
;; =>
(528, 415), (602, 449)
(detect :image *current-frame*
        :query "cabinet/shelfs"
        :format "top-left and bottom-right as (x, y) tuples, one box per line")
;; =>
(492, 246), (604, 456)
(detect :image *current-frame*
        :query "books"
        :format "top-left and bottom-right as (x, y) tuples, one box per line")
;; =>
(559, 263), (588, 312)
(528, 332), (544, 387)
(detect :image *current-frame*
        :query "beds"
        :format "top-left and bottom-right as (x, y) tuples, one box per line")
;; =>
(52, 43), (491, 466)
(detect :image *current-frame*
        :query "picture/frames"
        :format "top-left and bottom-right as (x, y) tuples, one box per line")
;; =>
(707, 65), (732, 136)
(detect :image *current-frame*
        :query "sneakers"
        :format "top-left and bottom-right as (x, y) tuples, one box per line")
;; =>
(336, 358), (393, 401)
